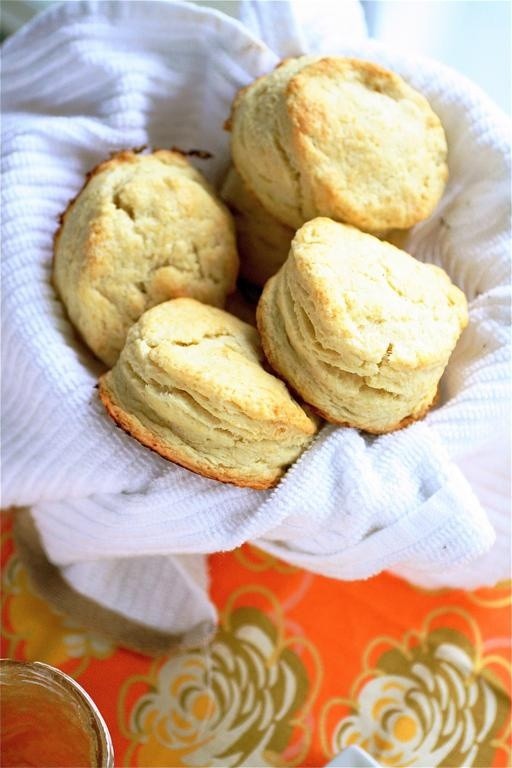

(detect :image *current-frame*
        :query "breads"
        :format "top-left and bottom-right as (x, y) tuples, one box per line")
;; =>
(214, 158), (300, 291)
(50, 144), (239, 372)
(256, 215), (473, 434)
(98, 296), (319, 491)
(220, 49), (453, 236)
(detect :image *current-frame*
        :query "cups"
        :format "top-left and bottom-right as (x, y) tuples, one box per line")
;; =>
(0, 657), (116, 768)
